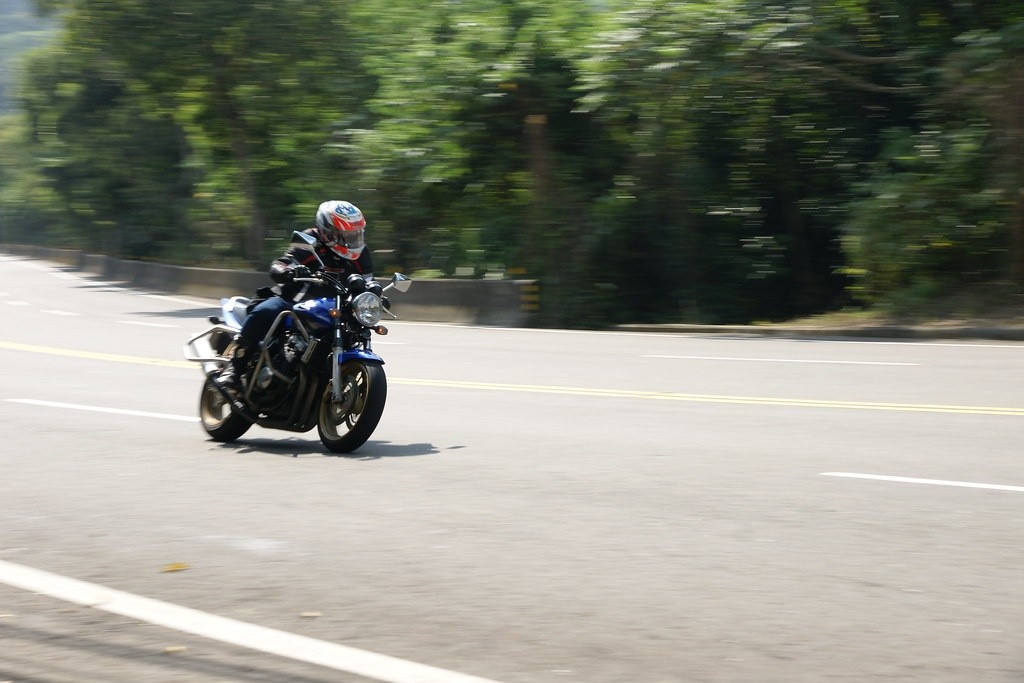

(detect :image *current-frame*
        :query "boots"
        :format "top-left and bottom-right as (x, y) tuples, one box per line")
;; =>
(215, 332), (256, 390)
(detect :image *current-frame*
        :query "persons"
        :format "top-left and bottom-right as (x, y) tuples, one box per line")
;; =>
(232, 200), (373, 368)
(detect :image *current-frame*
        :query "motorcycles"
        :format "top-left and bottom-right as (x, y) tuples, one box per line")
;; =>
(184, 229), (414, 455)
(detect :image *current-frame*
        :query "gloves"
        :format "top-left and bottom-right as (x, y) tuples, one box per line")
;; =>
(381, 295), (391, 314)
(280, 263), (312, 283)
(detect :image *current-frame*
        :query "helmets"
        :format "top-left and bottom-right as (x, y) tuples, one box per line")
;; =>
(315, 200), (366, 260)
